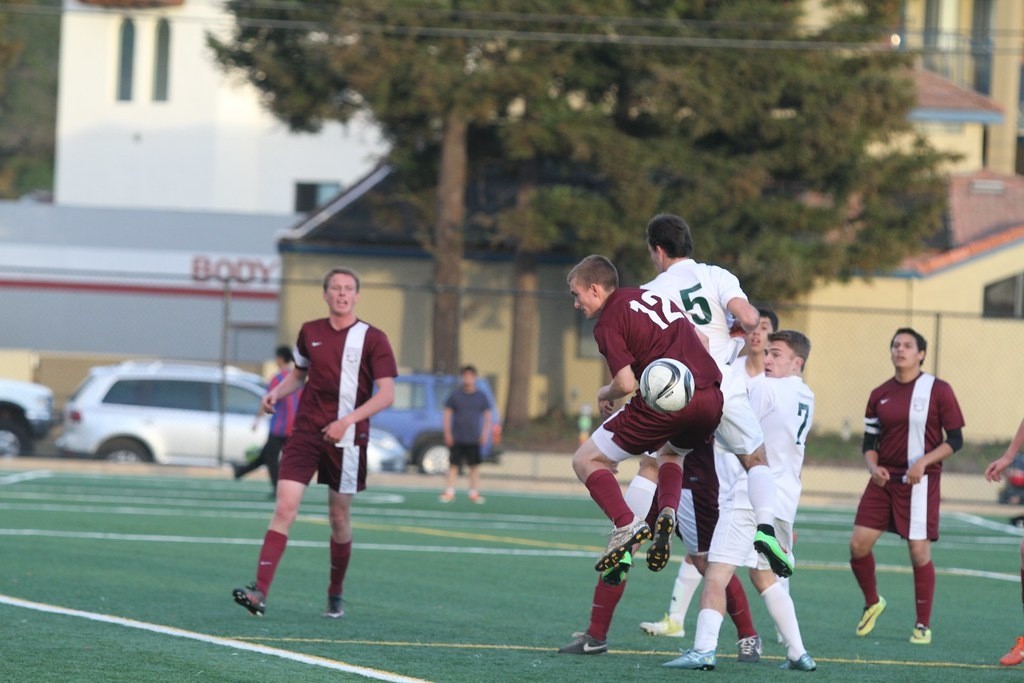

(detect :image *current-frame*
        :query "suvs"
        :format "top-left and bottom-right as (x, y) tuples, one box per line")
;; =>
(0, 380), (71, 461)
(54, 357), (413, 478)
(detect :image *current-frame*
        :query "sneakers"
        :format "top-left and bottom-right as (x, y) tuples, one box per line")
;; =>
(779, 653), (816, 672)
(753, 530), (794, 578)
(661, 648), (716, 671)
(640, 613), (686, 638)
(645, 506), (679, 571)
(1000, 636), (1024, 665)
(594, 517), (652, 572)
(856, 595), (887, 636)
(558, 630), (608, 655)
(322, 594), (346, 618)
(774, 628), (784, 643)
(910, 627), (932, 644)
(734, 634), (763, 664)
(600, 550), (634, 586)
(232, 582), (266, 617)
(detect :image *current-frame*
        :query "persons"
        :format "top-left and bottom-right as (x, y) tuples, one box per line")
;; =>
(440, 366), (490, 503)
(639, 308), (784, 643)
(601, 212), (795, 585)
(232, 269), (399, 616)
(558, 449), (764, 661)
(664, 329), (816, 669)
(985, 418), (1024, 665)
(851, 327), (965, 643)
(230, 345), (305, 496)
(568, 254), (724, 572)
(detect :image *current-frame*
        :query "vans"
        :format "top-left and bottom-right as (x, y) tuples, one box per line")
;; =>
(365, 370), (504, 475)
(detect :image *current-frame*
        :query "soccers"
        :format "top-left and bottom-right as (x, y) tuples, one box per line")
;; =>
(639, 356), (696, 414)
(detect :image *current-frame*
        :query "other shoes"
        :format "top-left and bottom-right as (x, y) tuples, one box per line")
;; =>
(439, 493), (455, 501)
(470, 492), (486, 504)
(229, 461), (244, 481)
(269, 493), (277, 500)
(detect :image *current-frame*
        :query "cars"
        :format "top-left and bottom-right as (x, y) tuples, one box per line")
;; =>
(999, 452), (1024, 507)
